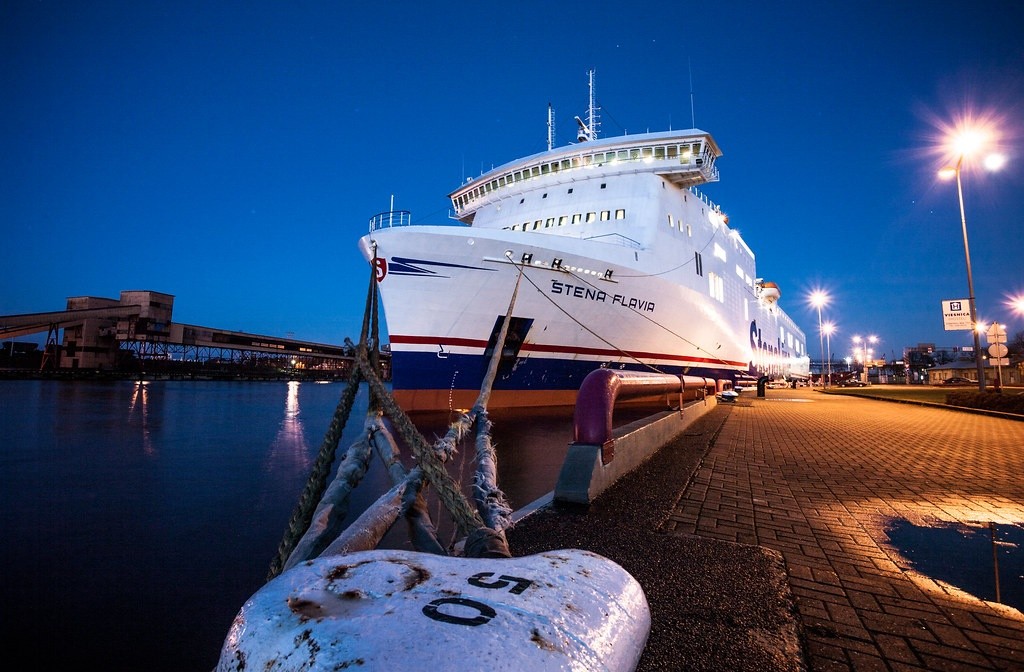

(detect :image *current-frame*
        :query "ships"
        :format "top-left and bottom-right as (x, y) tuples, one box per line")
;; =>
(357, 68), (810, 545)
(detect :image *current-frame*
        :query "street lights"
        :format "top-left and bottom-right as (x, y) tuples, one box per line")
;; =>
(936, 119), (999, 394)
(852, 334), (880, 383)
(845, 357), (852, 372)
(822, 324), (833, 386)
(813, 294), (828, 388)
(973, 322), (1004, 392)
